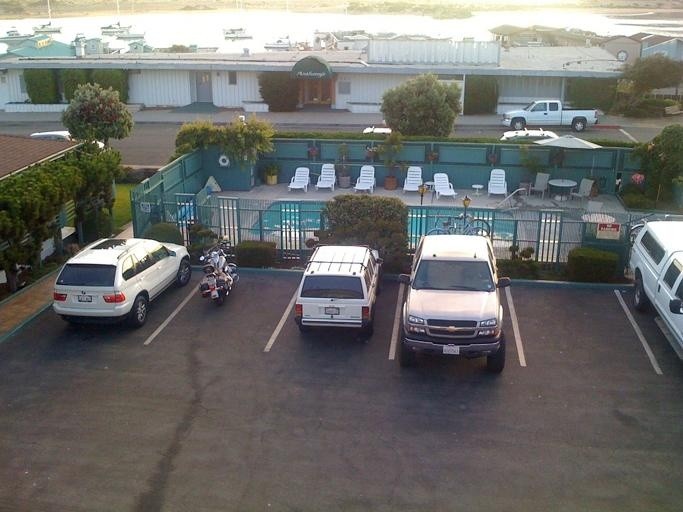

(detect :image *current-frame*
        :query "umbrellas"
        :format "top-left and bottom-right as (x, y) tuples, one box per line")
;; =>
(533, 135), (603, 201)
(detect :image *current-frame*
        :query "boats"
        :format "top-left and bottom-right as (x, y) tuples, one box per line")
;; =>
(2, 29), (35, 43)
(100, 24), (132, 34)
(222, 26), (245, 34)
(264, 42), (299, 51)
(225, 34), (252, 40)
(117, 32), (145, 40)
(33, 23), (63, 33)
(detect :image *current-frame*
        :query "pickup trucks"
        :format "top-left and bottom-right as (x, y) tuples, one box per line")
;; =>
(501, 98), (599, 131)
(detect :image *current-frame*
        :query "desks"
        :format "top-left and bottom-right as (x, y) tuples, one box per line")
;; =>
(471, 183), (484, 198)
(580, 214), (615, 231)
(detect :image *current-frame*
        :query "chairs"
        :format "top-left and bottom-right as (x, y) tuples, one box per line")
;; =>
(587, 200), (604, 215)
(518, 172), (594, 201)
(487, 170), (508, 198)
(354, 165), (376, 193)
(288, 162), (337, 193)
(403, 165), (458, 201)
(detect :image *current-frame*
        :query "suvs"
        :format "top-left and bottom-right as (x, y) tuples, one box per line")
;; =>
(52, 236), (193, 328)
(396, 233), (512, 371)
(294, 242), (383, 330)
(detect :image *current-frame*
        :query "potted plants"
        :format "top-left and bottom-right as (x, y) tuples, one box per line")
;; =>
(424, 147), (439, 161)
(338, 164), (353, 189)
(262, 162), (282, 185)
(306, 142), (378, 160)
(377, 130), (402, 190)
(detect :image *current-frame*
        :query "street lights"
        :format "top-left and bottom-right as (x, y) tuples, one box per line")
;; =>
(418, 183), (428, 237)
(461, 194), (472, 227)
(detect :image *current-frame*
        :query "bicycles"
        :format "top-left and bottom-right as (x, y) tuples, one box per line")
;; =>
(426, 213), (490, 237)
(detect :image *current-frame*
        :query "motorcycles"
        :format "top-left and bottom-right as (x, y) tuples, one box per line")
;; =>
(198, 234), (242, 306)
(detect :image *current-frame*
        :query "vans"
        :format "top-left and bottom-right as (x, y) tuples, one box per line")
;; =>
(628, 219), (681, 365)
(498, 128), (558, 142)
(30, 130), (112, 154)
